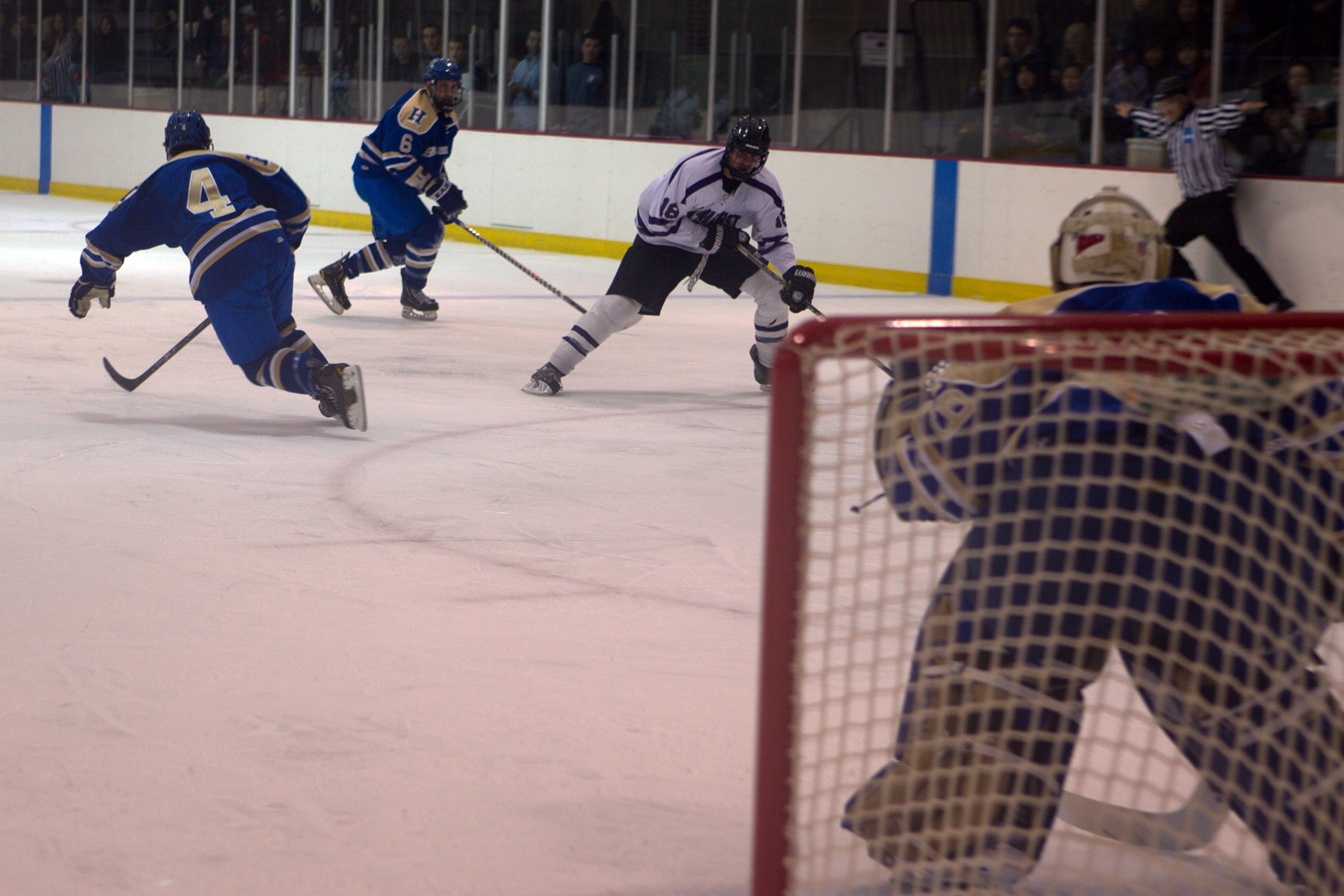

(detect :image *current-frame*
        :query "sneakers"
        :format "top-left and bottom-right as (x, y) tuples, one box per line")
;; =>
(308, 251), (351, 315)
(749, 344), (774, 391)
(315, 363), (367, 432)
(521, 361), (565, 395)
(400, 282), (439, 321)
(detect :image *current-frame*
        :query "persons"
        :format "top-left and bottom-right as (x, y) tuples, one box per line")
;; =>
(996, 17), (1050, 92)
(506, 27), (561, 128)
(520, 112), (817, 399)
(306, 59), (469, 323)
(1113, 74), (1296, 314)
(840, 188), (1344, 896)
(66, 107), (367, 432)
(564, 32), (609, 136)
(9, 0), (1344, 179)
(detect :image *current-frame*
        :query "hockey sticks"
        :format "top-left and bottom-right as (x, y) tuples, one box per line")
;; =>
(733, 240), (899, 380)
(1054, 774), (1229, 849)
(102, 316), (212, 392)
(452, 217), (644, 334)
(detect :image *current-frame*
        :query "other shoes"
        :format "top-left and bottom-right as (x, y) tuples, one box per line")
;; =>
(1269, 297), (1293, 312)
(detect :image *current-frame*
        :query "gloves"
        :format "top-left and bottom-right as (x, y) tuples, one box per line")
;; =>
(779, 265), (816, 313)
(68, 275), (116, 318)
(700, 223), (750, 254)
(426, 177), (468, 221)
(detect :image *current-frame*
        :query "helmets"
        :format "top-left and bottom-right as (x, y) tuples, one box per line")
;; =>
(423, 58), (464, 112)
(1151, 76), (1188, 102)
(725, 115), (771, 178)
(1049, 195), (1172, 293)
(163, 110), (212, 155)
